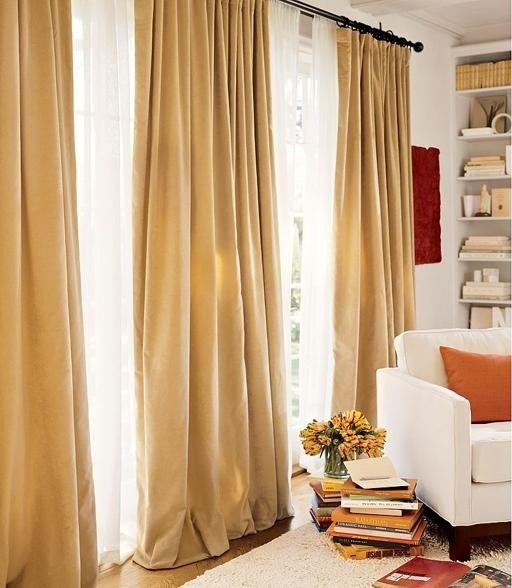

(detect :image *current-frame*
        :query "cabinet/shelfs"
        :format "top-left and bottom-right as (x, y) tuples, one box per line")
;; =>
(454, 48), (510, 306)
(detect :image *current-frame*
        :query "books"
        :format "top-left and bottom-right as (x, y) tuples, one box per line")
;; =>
(445, 565), (512, 588)
(459, 142), (510, 303)
(308, 459), (427, 558)
(373, 555), (472, 588)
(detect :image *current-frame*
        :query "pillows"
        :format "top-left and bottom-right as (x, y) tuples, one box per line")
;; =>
(438, 344), (511, 424)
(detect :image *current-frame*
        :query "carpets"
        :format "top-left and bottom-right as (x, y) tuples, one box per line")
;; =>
(175, 502), (510, 587)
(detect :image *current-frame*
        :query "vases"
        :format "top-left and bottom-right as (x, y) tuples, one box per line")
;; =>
(320, 443), (356, 480)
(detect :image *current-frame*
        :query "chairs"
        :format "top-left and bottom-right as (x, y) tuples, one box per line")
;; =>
(375, 326), (511, 562)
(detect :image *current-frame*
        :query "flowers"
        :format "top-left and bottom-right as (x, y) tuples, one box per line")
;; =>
(298, 409), (388, 473)
